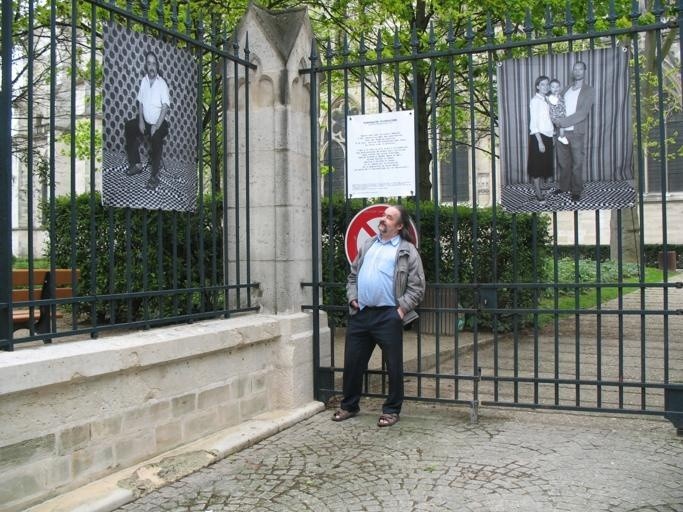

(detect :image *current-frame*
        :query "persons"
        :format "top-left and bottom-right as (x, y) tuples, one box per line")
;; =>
(123, 51), (171, 191)
(329, 204), (426, 427)
(553, 61), (596, 203)
(546, 79), (574, 145)
(525, 76), (556, 205)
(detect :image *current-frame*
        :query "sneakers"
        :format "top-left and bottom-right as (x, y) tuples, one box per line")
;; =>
(534, 185), (552, 201)
(552, 187), (582, 202)
(124, 166), (142, 176)
(557, 136), (569, 145)
(147, 177), (160, 189)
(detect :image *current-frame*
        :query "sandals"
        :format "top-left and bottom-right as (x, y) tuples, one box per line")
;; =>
(377, 413), (398, 426)
(330, 405), (362, 422)
